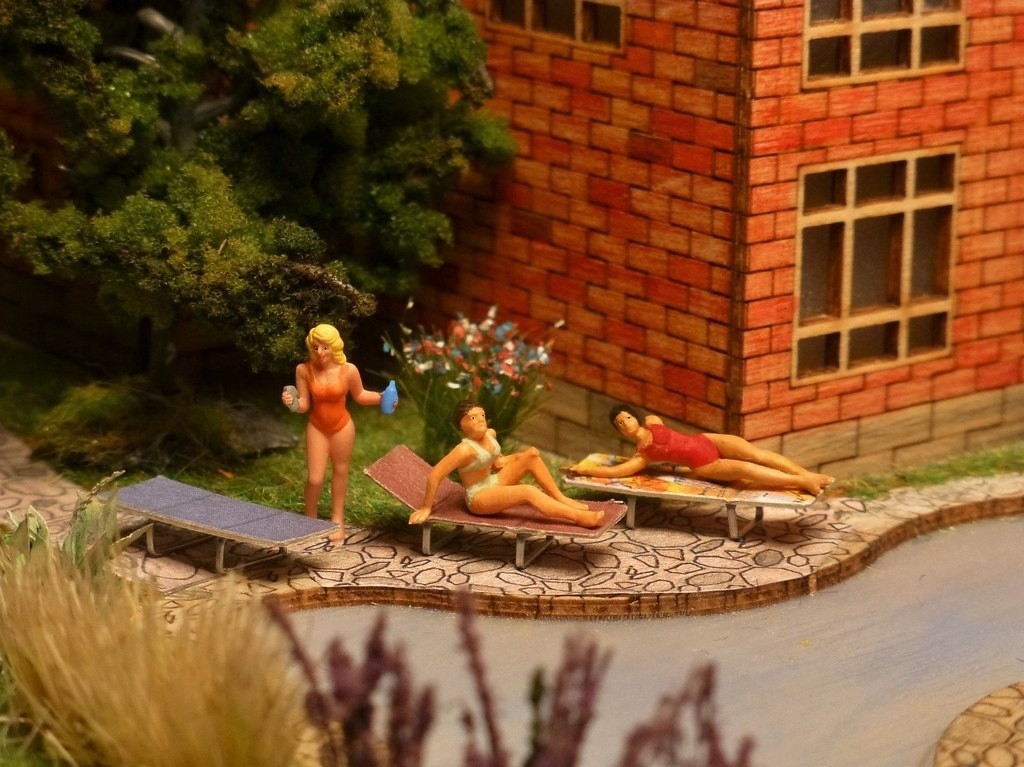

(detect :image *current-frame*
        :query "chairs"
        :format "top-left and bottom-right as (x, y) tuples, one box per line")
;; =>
(565, 449), (822, 544)
(93, 471), (342, 575)
(365, 444), (630, 570)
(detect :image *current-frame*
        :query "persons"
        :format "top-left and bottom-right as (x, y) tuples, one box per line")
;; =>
(409, 400), (604, 529)
(560, 404), (836, 498)
(282, 324), (398, 541)
